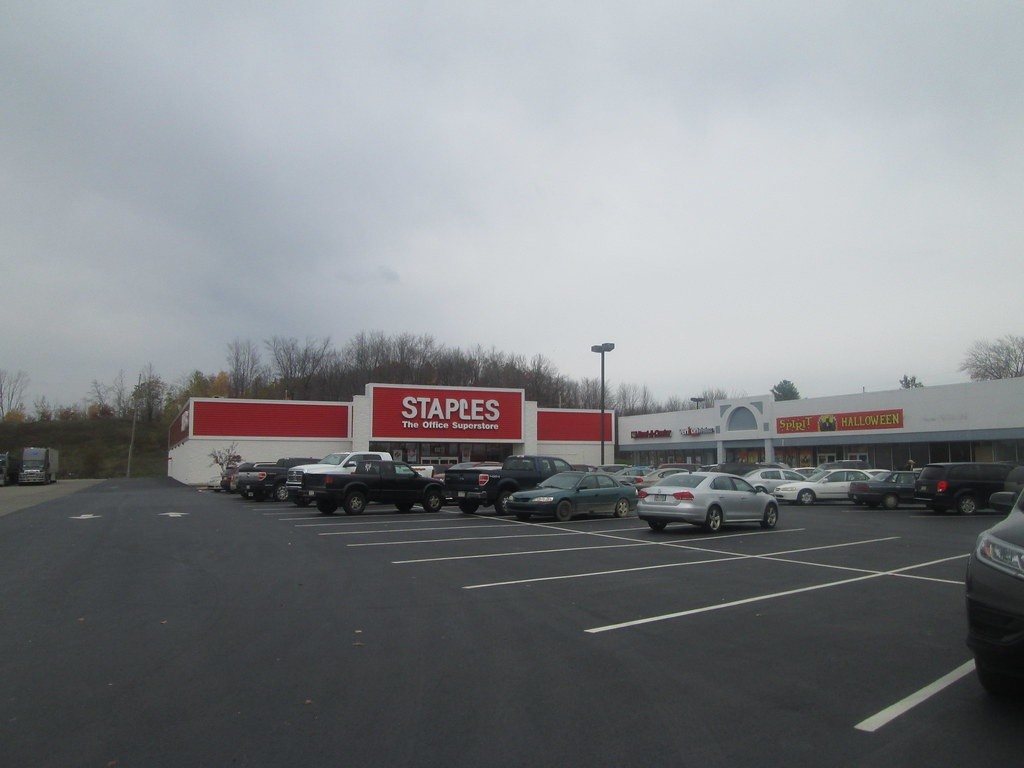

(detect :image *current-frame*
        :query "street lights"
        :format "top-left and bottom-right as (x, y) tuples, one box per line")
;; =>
(592, 343), (615, 465)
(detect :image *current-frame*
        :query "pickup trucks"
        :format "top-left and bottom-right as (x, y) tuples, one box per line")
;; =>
(444, 455), (577, 516)
(299, 461), (447, 515)
(285, 451), (433, 506)
(238, 457), (323, 502)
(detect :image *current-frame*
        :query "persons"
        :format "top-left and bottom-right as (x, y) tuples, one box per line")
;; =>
(903, 459), (916, 471)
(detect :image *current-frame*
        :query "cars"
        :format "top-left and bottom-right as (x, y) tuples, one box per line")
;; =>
(556, 464), (632, 478)
(636, 472), (779, 534)
(613, 467), (654, 485)
(506, 471), (638, 522)
(793, 462), (905, 483)
(965, 487), (1024, 698)
(847, 471), (926, 510)
(633, 468), (694, 491)
(208, 462), (277, 499)
(773, 468), (874, 505)
(659, 463), (788, 479)
(733, 468), (808, 494)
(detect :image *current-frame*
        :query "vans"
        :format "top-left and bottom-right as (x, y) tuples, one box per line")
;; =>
(913, 462), (1024, 515)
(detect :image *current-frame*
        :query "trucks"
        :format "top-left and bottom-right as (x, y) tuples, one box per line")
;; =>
(0, 451), (19, 486)
(17, 447), (59, 484)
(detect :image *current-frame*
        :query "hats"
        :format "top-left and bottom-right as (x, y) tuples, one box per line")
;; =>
(907, 460), (915, 464)
